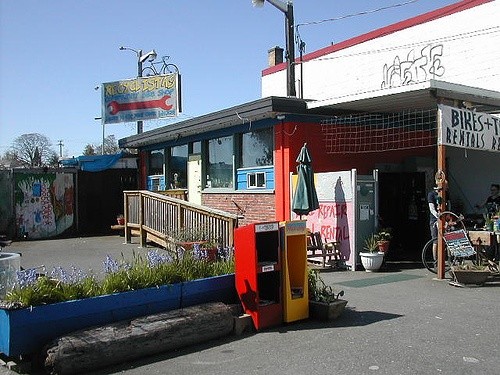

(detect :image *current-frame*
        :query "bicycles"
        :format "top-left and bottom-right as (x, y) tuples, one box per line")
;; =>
(421, 221), (483, 274)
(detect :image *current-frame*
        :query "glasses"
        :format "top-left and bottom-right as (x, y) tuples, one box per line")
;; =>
(490, 189), (497, 191)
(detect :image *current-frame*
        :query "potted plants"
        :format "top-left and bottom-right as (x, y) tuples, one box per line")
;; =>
(357, 233), (390, 273)
(451, 263), (492, 285)
(117, 213), (124, 224)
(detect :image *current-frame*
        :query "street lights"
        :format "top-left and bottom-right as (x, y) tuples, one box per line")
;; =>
(118, 46), (157, 135)
(251, 0), (296, 97)
(94, 84), (104, 156)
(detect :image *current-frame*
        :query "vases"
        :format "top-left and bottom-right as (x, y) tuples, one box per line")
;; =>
(311, 298), (349, 321)
(1, 281), (183, 359)
(200, 247), (217, 261)
(177, 239), (208, 250)
(181, 274), (237, 308)
(378, 238), (390, 253)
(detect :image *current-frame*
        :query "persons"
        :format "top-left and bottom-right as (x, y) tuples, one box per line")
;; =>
(428, 179), (451, 271)
(483, 183), (500, 262)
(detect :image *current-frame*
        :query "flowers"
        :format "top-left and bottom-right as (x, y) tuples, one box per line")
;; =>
(305, 268), (344, 302)
(4, 222), (236, 309)
(379, 228), (391, 240)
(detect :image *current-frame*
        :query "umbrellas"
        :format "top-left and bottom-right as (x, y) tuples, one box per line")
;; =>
(292, 142), (320, 220)
